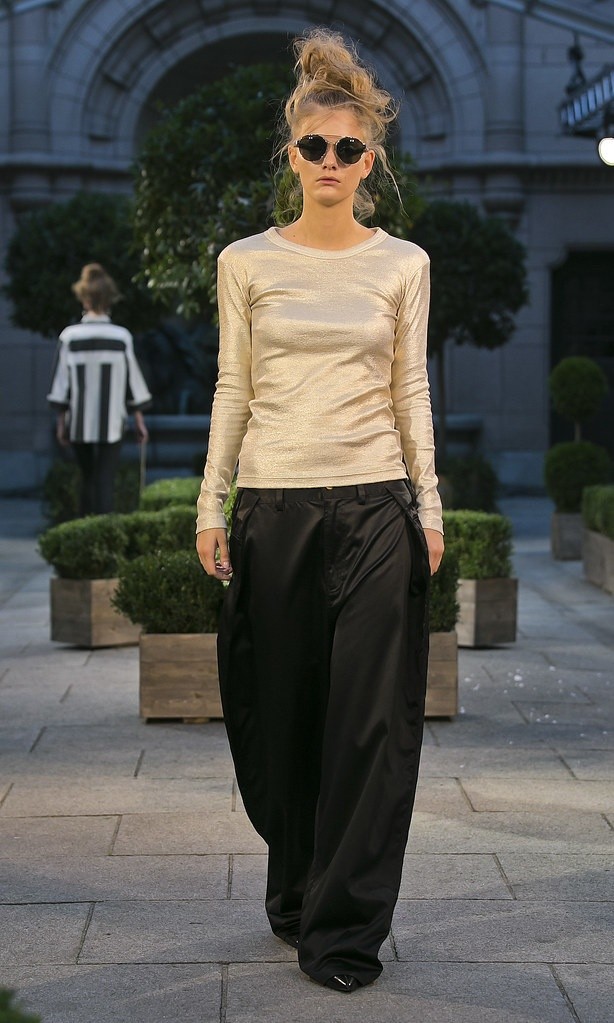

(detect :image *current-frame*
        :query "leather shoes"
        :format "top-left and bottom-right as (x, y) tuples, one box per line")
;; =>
(284, 933), (300, 947)
(327, 976), (358, 992)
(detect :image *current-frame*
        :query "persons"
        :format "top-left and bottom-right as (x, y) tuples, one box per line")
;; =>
(47, 264), (151, 513)
(195, 30), (445, 995)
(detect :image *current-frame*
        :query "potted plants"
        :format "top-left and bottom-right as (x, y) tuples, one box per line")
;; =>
(582, 486), (614, 595)
(543, 441), (610, 560)
(113, 546), (460, 724)
(443, 510), (517, 648)
(35, 515), (145, 647)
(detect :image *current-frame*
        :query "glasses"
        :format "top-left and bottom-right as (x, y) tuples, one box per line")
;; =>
(292, 134), (368, 166)
